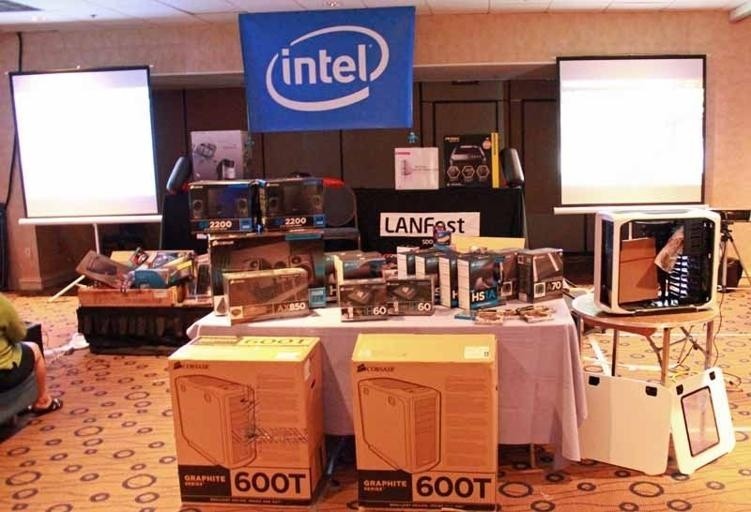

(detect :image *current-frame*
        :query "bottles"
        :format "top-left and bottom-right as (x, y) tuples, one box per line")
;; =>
(224, 160), (236, 179)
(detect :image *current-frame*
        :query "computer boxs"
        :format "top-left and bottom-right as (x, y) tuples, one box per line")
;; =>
(175, 375), (257, 469)
(357, 377), (441, 476)
(594, 207), (720, 315)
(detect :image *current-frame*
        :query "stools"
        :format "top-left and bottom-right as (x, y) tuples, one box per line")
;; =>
(1, 373), (38, 429)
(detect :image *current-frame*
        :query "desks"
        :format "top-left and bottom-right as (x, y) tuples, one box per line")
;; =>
(572, 293), (720, 387)
(185, 298), (587, 470)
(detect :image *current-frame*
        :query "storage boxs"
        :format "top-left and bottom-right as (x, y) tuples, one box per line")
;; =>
(77, 250), (194, 306)
(352, 333), (498, 510)
(168, 336), (326, 509)
(191, 130), (253, 180)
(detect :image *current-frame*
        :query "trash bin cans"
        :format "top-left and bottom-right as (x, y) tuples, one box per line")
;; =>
(718, 257), (743, 293)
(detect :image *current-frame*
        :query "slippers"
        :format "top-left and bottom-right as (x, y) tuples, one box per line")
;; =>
(31, 398), (64, 415)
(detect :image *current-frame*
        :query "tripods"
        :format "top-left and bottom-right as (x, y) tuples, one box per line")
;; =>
(718, 233), (751, 293)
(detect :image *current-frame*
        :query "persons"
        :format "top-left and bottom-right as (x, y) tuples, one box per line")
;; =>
(0, 295), (63, 413)
